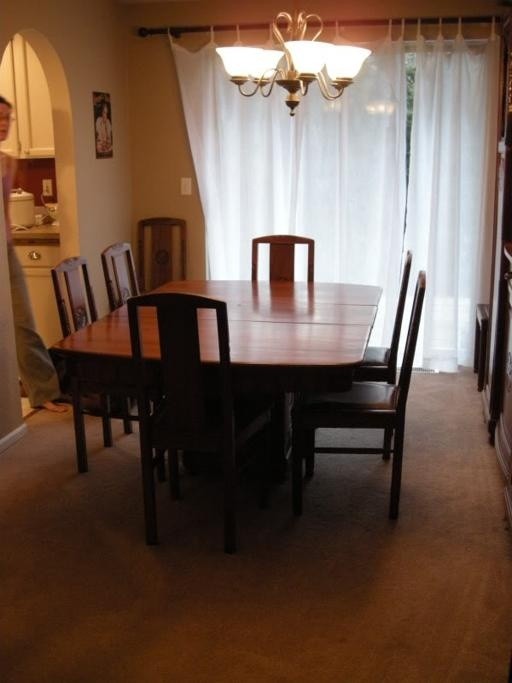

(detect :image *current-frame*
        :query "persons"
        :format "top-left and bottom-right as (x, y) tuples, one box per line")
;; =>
(0, 95), (68, 414)
(95, 102), (113, 154)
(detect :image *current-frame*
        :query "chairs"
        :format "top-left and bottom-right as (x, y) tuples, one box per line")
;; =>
(136, 217), (188, 293)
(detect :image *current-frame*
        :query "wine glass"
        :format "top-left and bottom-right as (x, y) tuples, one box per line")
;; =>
(44, 203), (58, 226)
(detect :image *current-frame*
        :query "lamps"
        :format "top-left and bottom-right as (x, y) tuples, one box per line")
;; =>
(213, 0), (374, 119)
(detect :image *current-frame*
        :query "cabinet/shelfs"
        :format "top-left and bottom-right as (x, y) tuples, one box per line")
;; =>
(10, 240), (61, 350)
(0, 31), (58, 161)
(481, 7), (512, 539)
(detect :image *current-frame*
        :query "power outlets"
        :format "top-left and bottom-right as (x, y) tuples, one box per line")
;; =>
(42, 179), (53, 197)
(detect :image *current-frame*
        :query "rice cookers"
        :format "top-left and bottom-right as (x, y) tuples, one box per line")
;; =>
(6, 189), (36, 228)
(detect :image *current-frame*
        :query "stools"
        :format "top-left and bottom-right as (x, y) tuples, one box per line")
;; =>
(472, 304), (491, 392)
